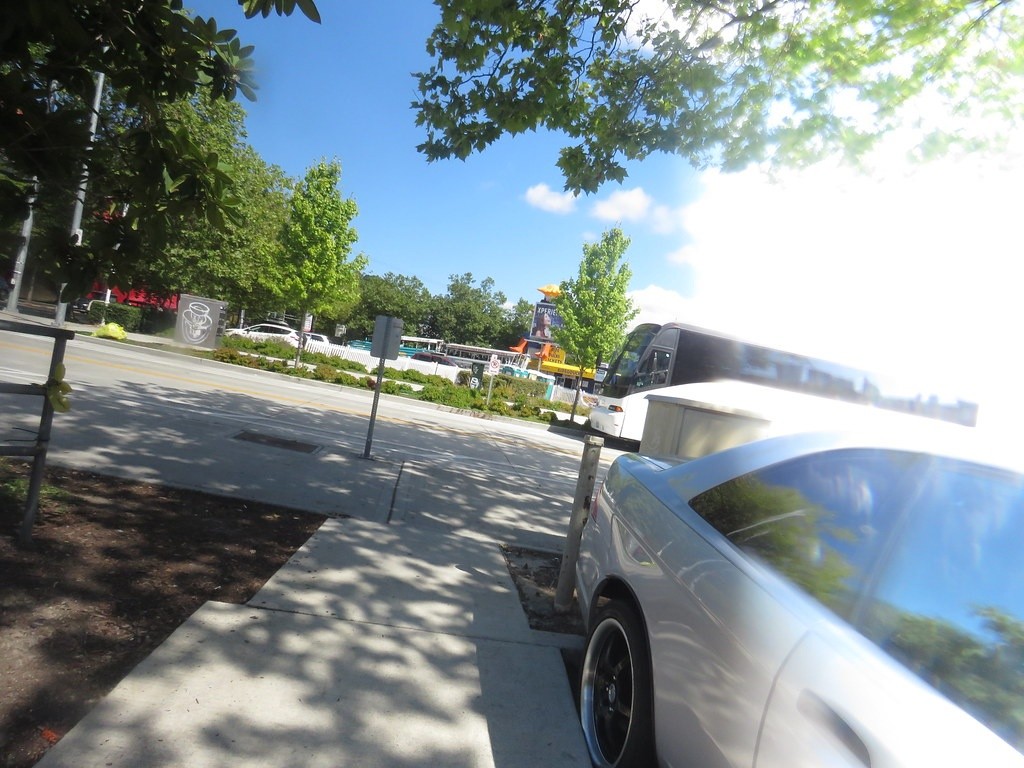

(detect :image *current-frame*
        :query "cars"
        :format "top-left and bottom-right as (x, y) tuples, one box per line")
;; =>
(302, 332), (329, 345)
(410, 351), (459, 368)
(223, 323), (304, 351)
(573, 430), (1024, 768)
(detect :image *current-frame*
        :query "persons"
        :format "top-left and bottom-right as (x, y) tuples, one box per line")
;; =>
(533, 313), (552, 339)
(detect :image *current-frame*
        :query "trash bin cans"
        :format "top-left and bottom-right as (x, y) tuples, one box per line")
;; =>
(502, 366), (556, 401)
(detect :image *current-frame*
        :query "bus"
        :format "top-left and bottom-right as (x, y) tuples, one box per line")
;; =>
(502, 364), (555, 401)
(346, 331), (444, 359)
(528, 360), (596, 395)
(445, 343), (530, 376)
(589, 317), (985, 447)
